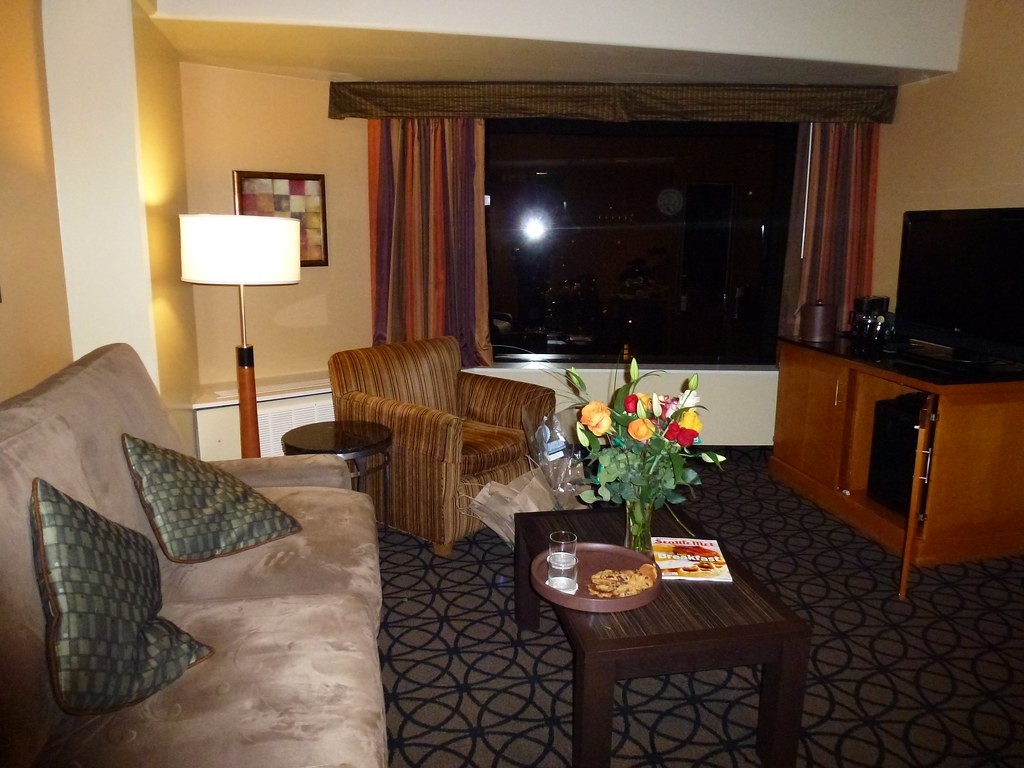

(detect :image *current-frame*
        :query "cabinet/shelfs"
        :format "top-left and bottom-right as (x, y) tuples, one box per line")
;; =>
(770, 329), (1024, 601)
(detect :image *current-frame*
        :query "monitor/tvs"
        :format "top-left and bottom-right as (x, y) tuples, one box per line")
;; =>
(895, 208), (1024, 373)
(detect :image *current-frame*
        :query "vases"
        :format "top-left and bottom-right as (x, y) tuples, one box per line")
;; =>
(622, 503), (654, 560)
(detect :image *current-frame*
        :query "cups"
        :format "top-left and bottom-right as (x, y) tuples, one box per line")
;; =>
(547, 554), (579, 590)
(549, 532), (577, 566)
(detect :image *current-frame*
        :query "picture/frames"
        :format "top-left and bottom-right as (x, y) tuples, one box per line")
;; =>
(233, 168), (329, 266)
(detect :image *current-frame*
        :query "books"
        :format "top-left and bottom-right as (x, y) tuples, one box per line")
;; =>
(651, 535), (734, 586)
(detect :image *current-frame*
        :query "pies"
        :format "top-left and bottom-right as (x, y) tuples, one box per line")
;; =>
(588, 569), (652, 598)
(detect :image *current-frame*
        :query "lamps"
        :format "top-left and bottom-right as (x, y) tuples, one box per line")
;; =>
(180, 214), (302, 286)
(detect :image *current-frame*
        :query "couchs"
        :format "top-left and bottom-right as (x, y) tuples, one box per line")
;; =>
(327, 334), (555, 552)
(0, 342), (387, 768)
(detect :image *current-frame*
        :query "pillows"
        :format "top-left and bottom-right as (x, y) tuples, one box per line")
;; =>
(120, 432), (302, 564)
(30, 476), (216, 718)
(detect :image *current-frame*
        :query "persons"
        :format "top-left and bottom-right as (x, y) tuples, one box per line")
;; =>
(613, 246), (672, 299)
(510, 200), (553, 331)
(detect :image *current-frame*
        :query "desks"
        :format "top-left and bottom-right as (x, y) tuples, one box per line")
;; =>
(281, 419), (392, 529)
(514, 505), (814, 768)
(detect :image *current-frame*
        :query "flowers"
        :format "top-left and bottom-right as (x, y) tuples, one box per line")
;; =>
(554, 345), (730, 503)
